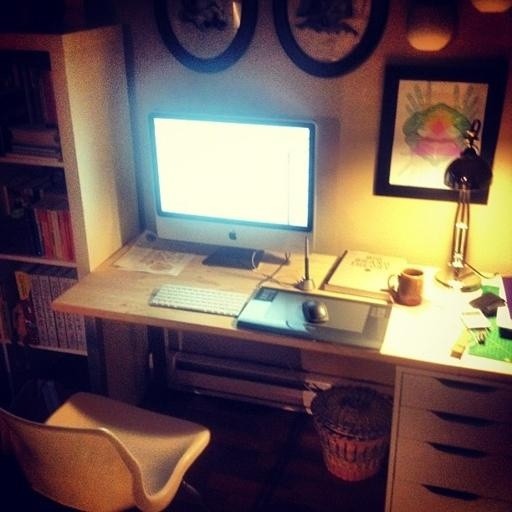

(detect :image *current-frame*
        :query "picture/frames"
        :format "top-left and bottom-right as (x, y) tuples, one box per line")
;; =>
(373, 63), (509, 206)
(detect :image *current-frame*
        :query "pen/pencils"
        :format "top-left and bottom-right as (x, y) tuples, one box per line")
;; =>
(324, 250), (348, 284)
(305, 236), (309, 279)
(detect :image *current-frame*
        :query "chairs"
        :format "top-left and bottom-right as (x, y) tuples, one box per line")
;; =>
(0, 389), (210, 511)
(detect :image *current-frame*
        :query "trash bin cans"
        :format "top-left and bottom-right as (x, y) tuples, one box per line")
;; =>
(311, 384), (394, 482)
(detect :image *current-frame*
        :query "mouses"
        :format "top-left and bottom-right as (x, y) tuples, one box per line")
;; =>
(303, 298), (330, 323)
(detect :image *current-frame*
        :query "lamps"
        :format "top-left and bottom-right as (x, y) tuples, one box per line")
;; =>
(435, 120), (494, 291)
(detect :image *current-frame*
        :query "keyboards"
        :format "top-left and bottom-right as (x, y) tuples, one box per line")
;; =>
(150, 284), (249, 316)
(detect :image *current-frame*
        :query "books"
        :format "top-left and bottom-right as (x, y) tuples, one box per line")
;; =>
(323, 248), (411, 303)
(0, 62), (87, 357)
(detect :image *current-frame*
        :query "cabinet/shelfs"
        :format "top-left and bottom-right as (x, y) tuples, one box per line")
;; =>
(1, 27), (130, 399)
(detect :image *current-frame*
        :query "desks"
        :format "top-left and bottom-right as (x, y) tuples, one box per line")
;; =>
(50, 229), (510, 512)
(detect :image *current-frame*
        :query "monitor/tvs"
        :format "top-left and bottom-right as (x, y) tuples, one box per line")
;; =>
(147, 111), (318, 271)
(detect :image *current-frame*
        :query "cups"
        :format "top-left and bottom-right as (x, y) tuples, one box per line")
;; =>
(387, 268), (424, 306)
